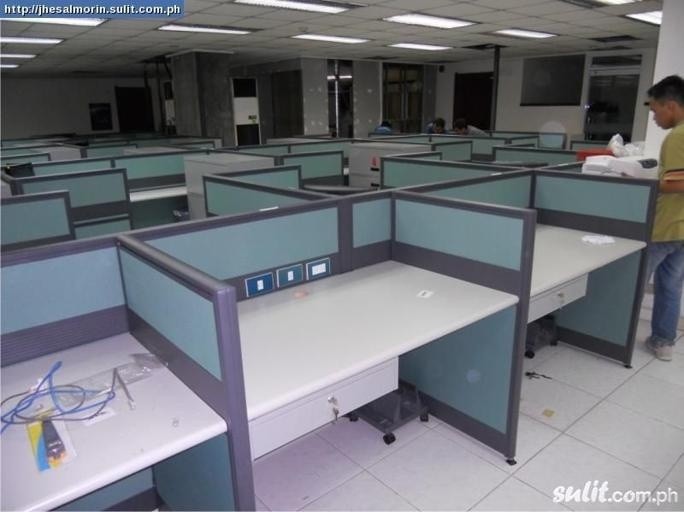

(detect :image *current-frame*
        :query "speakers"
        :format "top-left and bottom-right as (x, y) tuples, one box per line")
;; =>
(439, 64), (445, 73)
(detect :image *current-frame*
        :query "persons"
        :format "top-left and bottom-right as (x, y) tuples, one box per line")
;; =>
(619, 71), (684, 363)
(451, 118), (488, 136)
(426, 118), (448, 133)
(374, 118), (394, 135)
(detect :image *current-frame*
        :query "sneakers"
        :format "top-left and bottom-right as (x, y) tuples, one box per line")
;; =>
(645, 336), (673, 361)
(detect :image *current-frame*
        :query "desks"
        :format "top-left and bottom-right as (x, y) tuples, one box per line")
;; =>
(127, 186), (189, 203)
(236, 259), (520, 461)
(520, 221), (648, 323)
(0, 331), (236, 512)
